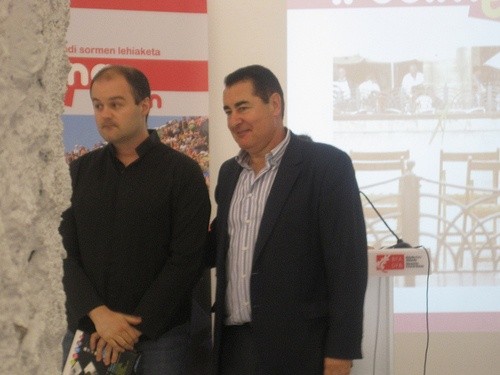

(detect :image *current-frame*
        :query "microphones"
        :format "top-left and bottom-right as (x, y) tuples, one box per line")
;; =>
(358, 189), (413, 249)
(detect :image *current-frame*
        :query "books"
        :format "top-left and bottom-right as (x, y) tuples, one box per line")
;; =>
(63, 329), (142, 375)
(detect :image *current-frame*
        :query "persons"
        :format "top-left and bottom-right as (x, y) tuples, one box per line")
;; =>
(334, 63), (500, 116)
(58, 66), (212, 375)
(205, 65), (368, 375)
